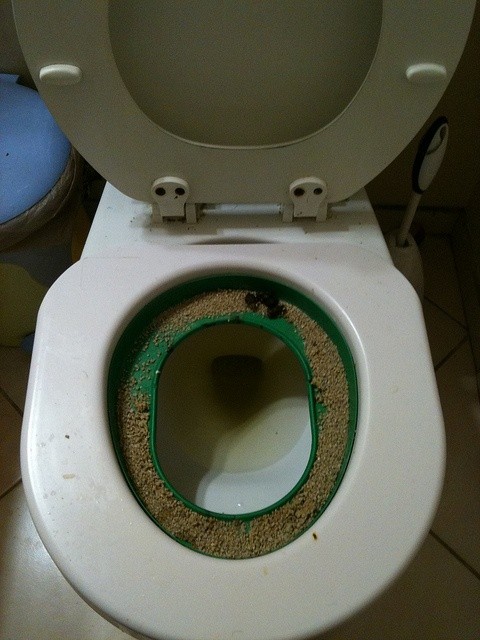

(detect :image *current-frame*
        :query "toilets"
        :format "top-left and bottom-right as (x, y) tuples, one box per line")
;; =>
(13, 0), (477, 640)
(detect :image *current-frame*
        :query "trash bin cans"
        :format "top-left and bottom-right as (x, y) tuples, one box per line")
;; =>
(0, 75), (77, 348)
